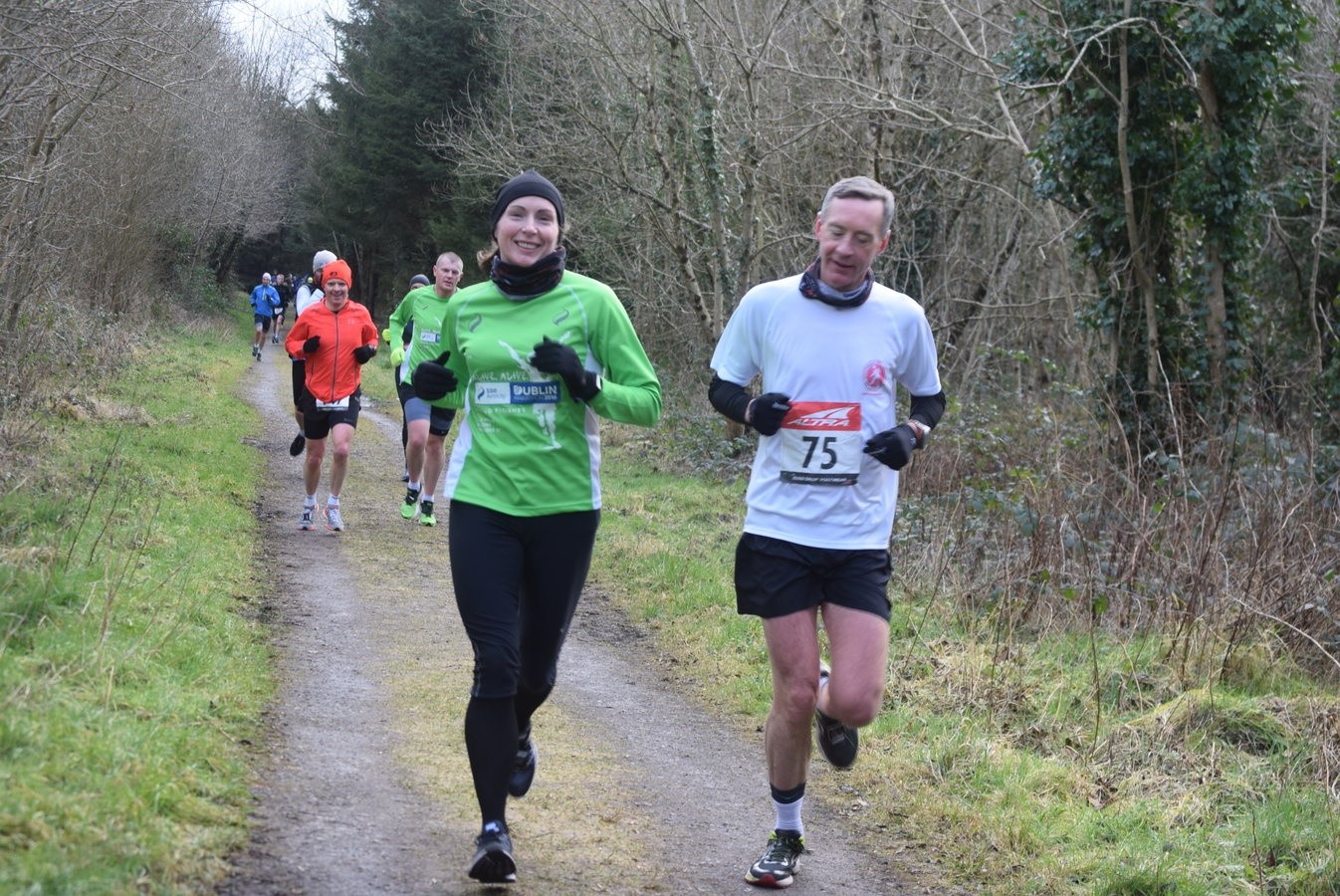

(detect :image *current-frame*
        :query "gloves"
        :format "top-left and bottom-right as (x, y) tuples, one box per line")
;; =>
(354, 346), (372, 363)
(863, 424), (919, 470)
(389, 348), (406, 368)
(303, 335), (320, 353)
(265, 294), (271, 301)
(530, 335), (591, 399)
(749, 392), (792, 437)
(251, 303), (256, 307)
(413, 351), (458, 402)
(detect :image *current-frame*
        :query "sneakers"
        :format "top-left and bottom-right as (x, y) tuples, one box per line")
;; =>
(297, 503), (319, 530)
(418, 500), (436, 527)
(401, 482), (422, 519)
(323, 504), (344, 532)
(745, 829), (805, 887)
(815, 664), (860, 770)
(468, 820), (516, 883)
(507, 739), (539, 797)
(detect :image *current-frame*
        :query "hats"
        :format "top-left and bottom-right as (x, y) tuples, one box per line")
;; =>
(313, 250), (337, 274)
(409, 274), (430, 287)
(321, 259), (351, 290)
(491, 170), (565, 243)
(262, 273), (271, 280)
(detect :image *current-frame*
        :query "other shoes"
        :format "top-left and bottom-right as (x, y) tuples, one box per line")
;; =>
(252, 347), (258, 357)
(402, 469), (409, 482)
(272, 335), (279, 344)
(257, 353), (261, 361)
(290, 433), (305, 456)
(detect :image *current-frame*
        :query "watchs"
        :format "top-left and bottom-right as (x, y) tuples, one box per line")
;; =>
(910, 420), (931, 449)
(582, 374), (602, 404)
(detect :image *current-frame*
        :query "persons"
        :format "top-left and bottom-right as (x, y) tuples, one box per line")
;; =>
(705, 172), (946, 888)
(381, 273), (428, 484)
(284, 258), (376, 535)
(270, 275), (288, 344)
(399, 169), (663, 892)
(271, 270), (314, 322)
(389, 251), (464, 529)
(289, 251), (338, 457)
(249, 273), (280, 361)
